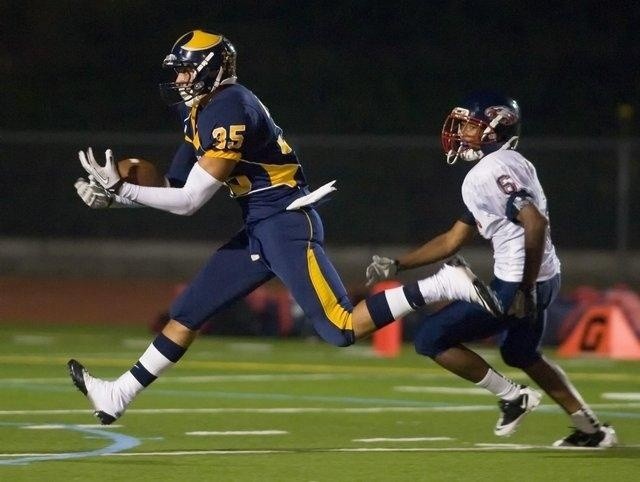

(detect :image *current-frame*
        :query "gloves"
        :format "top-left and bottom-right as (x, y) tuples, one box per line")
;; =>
(506, 284), (538, 320)
(74, 174), (115, 210)
(365, 255), (398, 286)
(78, 147), (125, 193)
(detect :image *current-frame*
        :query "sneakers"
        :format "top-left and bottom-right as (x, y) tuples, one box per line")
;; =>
(67, 359), (125, 425)
(441, 253), (504, 320)
(494, 385), (542, 436)
(552, 425), (618, 449)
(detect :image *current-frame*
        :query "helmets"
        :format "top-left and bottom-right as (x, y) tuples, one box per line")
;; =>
(157, 30), (237, 106)
(441, 88), (522, 164)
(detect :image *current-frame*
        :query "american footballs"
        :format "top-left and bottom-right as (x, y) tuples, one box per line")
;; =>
(116, 158), (166, 188)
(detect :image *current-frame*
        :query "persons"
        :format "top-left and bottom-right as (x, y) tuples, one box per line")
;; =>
(364, 92), (619, 449)
(68, 28), (504, 425)
